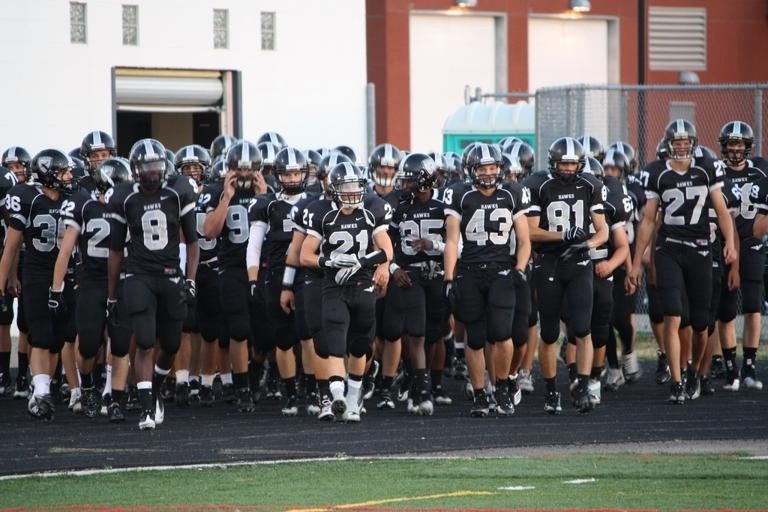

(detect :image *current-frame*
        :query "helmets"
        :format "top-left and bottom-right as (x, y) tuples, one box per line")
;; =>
(302, 149), (323, 192)
(175, 144), (212, 178)
(547, 137), (586, 185)
(460, 142), (484, 187)
(441, 150), (465, 185)
(210, 135), (239, 157)
(694, 144), (718, 161)
(393, 153), (437, 204)
(326, 161), (368, 204)
(272, 147), (309, 195)
(319, 152), (354, 180)
(663, 118), (699, 161)
(601, 148), (631, 174)
(499, 153), (523, 182)
(579, 155), (606, 178)
(718, 120), (755, 166)
(466, 143), (504, 189)
(67, 146), (90, 179)
(165, 148), (175, 180)
(257, 131), (287, 150)
(226, 142), (265, 198)
(502, 140), (536, 178)
(212, 159), (229, 180)
(79, 130), (116, 152)
(92, 158), (133, 193)
(578, 135), (605, 162)
(607, 140), (638, 167)
(128, 137), (168, 190)
(1, 146), (32, 182)
(424, 151), (448, 189)
(334, 145), (357, 163)
(367, 143), (404, 187)
(655, 137), (667, 160)
(257, 141), (282, 167)
(32, 148), (80, 199)
(497, 136), (523, 151)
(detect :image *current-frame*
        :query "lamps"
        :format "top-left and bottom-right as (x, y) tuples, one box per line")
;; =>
(570, 1), (593, 15)
(455, 0), (478, 8)
(677, 71), (701, 85)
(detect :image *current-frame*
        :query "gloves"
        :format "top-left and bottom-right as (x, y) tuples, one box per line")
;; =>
(246, 279), (266, 307)
(560, 242), (590, 263)
(439, 280), (461, 309)
(334, 256), (368, 286)
(178, 280), (197, 304)
(105, 298), (120, 327)
(47, 290), (69, 315)
(317, 253), (358, 269)
(564, 225), (587, 241)
(512, 269), (529, 288)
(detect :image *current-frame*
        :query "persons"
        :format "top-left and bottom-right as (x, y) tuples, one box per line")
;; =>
(1, 119), (768, 431)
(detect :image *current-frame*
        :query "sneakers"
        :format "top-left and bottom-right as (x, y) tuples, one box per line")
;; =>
(221, 382), (236, 403)
(266, 380), (283, 400)
(304, 392), (321, 416)
(430, 383), (453, 406)
(13, 376), (30, 399)
(740, 364), (764, 390)
(376, 386), (397, 410)
(80, 384), (100, 418)
(108, 399), (125, 422)
(470, 388), (491, 416)
(406, 384), (435, 417)
(68, 388), (81, 408)
(0, 371), (14, 398)
(317, 398), (335, 421)
(570, 377), (602, 405)
(99, 392), (112, 415)
(330, 393), (349, 424)
(453, 352), (468, 380)
(174, 381), (189, 408)
(669, 381), (686, 404)
(60, 384), (71, 402)
(621, 349), (642, 382)
(152, 390), (165, 424)
(508, 374), (522, 407)
(491, 384), (515, 416)
(236, 387), (255, 413)
(188, 386), (202, 405)
(342, 398), (361, 423)
(722, 366), (741, 392)
(575, 386), (595, 415)
(443, 349), (457, 379)
(199, 385), (215, 406)
(27, 390), (55, 422)
(710, 355), (724, 380)
(138, 408), (156, 430)
(281, 394), (299, 416)
(655, 349), (672, 383)
(543, 387), (563, 414)
(517, 369), (535, 393)
(362, 359), (380, 400)
(701, 376), (717, 394)
(606, 365), (626, 392)
(686, 373), (701, 400)
